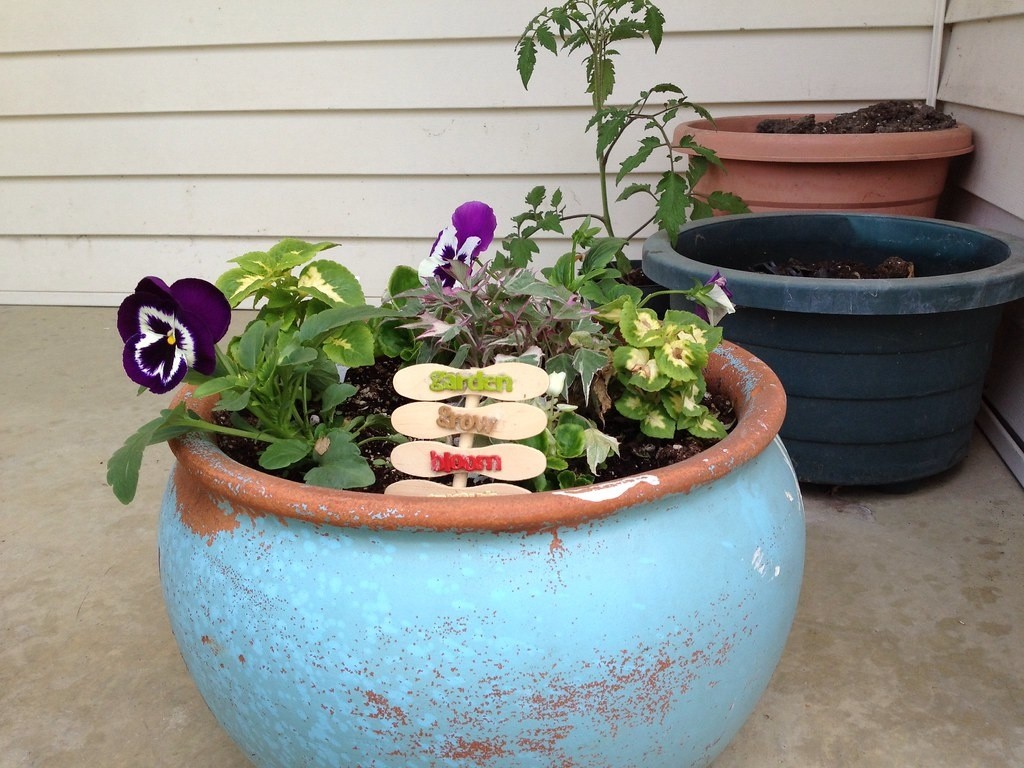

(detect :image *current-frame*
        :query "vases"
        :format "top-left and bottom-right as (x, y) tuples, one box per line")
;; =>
(639, 209), (1024, 491)
(668, 115), (977, 219)
(157, 338), (808, 768)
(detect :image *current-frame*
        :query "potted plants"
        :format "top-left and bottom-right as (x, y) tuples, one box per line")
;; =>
(489, 0), (755, 318)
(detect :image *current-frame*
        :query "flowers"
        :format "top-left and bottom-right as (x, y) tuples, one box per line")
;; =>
(106, 201), (755, 505)
